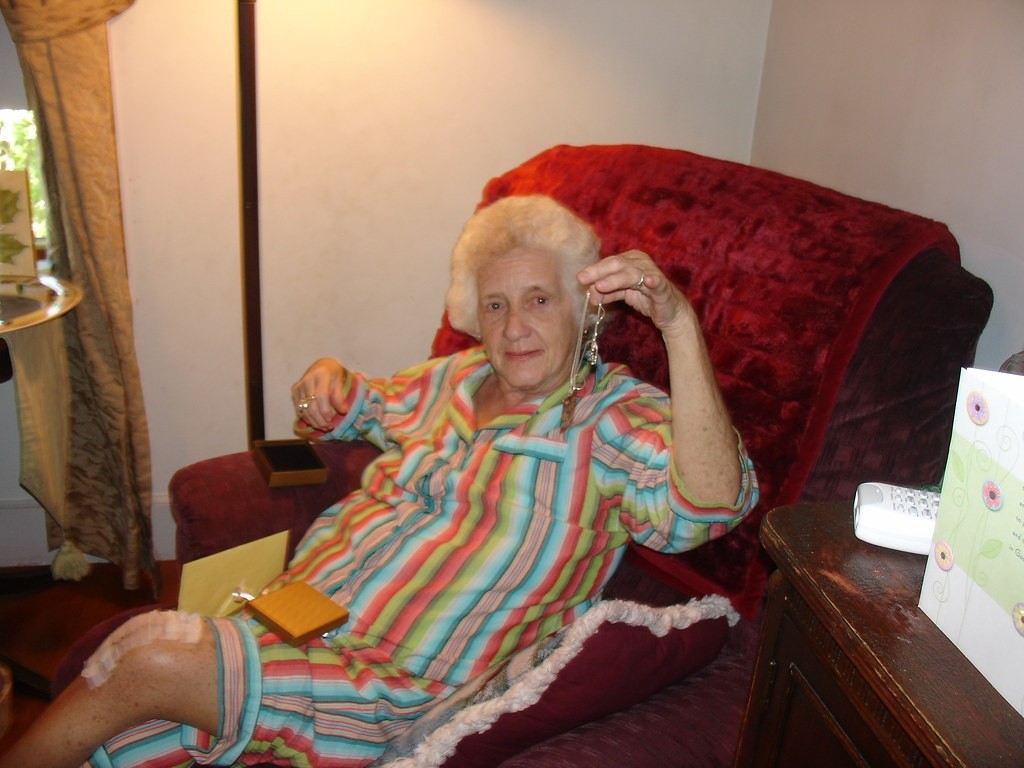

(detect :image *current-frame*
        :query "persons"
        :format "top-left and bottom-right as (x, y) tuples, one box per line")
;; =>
(0, 193), (758, 768)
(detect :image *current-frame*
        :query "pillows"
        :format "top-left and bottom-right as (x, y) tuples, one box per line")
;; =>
(365, 593), (741, 768)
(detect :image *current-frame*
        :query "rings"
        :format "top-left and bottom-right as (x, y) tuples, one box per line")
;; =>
(297, 395), (316, 409)
(631, 266), (645, 290)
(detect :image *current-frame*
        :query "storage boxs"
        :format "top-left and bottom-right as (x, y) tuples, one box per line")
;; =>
(251, 438), (328, 486)
(246, 580), (348, 647)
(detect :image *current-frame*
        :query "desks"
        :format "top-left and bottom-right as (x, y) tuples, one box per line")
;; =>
(0, 271), (83, 384)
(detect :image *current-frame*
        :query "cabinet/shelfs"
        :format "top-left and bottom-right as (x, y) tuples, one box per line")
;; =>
(729, 500), (1024, 768)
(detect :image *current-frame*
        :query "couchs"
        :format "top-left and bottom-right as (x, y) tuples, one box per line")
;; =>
(49, 144), (996, 768)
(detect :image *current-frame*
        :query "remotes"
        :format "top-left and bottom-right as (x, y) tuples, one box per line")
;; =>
(853, 483), (942, 555)
